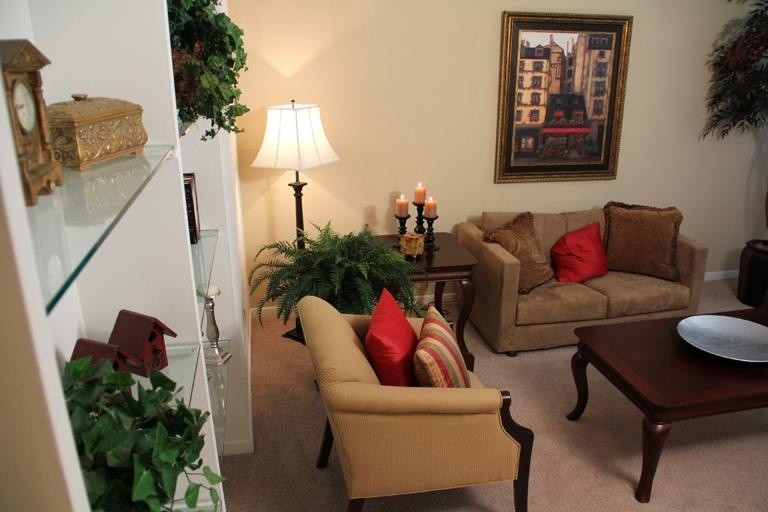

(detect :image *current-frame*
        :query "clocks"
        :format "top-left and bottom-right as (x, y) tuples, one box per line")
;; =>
(0, 39), (63, 206)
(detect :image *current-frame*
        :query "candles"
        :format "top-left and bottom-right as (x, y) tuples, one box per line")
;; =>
(396, 193), (409, 218)
(422, 196), (437, 218)
(414, 182), (426, 204)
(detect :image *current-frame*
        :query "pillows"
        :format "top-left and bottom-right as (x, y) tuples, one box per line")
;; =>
(551, 222), (609, 283)
(603, 201), (684, 281)
(365, 287), (418, 385)
(412, 303), (471, 388)
(482, 213), (566, 268)
(482, 212), (554, 295)
(561, 210), (606, 244)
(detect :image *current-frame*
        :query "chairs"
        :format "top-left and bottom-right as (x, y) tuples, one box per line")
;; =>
(295, 296), (534, 512)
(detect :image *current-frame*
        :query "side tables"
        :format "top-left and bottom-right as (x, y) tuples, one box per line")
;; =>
(371, 232), (479, 372)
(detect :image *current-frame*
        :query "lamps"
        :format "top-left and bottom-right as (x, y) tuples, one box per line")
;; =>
(249, 99), (341, 346)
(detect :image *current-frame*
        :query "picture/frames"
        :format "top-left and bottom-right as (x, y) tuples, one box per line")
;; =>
(493, 11), (633, 184)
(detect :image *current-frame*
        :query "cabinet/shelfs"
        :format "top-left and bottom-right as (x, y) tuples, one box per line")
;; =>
(0, 1), (257, 512)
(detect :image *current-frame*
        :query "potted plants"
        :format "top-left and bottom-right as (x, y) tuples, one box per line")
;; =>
(701, 0), (768, 308)
(166, 1), (249, 142)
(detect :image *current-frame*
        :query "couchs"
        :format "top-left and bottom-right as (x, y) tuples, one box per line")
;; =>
(453, 210), (709, 357)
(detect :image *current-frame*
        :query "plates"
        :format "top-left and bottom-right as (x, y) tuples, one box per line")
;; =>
(678, 312), (768, 365)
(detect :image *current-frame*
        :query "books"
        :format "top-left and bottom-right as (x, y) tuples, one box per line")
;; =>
(183, 173), (200, 244)
